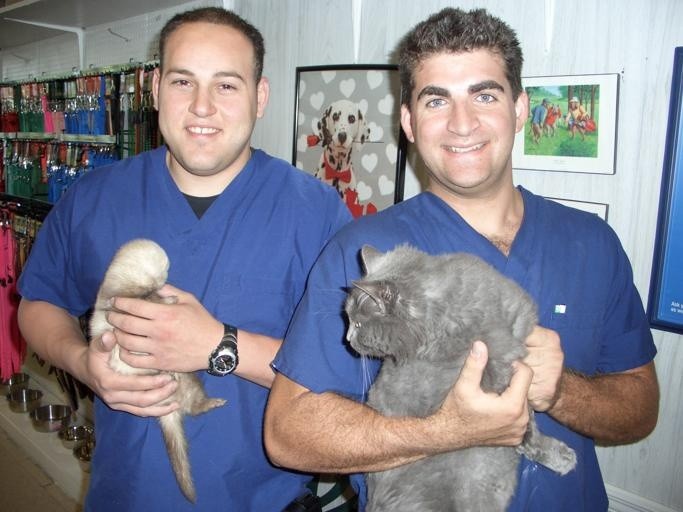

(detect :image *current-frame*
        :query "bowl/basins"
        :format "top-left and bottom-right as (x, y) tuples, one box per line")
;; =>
(0, 373), (96, 472)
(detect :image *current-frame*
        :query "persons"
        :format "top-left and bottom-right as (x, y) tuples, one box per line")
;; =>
(13, 7), (356, 512)
(264, 5), (660, 512)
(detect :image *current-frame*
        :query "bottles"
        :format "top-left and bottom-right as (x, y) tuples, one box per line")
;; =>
(1, 94), (118, 206)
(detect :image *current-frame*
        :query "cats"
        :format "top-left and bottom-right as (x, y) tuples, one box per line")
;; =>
(88, 238), (228, 505)
(341, 240), (578, 512)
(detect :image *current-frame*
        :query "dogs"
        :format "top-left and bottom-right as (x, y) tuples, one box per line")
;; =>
(311, 99), (372, 205)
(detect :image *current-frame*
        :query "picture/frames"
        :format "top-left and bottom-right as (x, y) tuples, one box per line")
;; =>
(506, 71), (619, 176)
(292, 64), (413, 223)
(531, 195), (611, 225)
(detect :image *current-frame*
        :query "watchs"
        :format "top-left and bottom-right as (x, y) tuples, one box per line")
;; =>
(205, 321), (238, 376)
